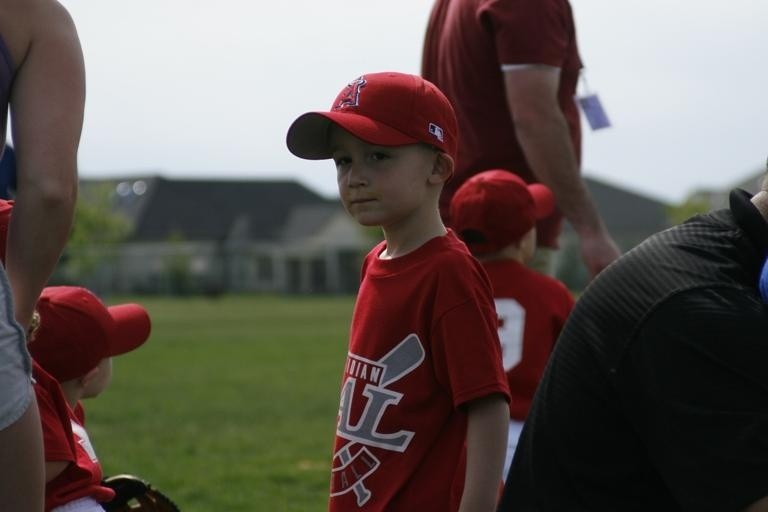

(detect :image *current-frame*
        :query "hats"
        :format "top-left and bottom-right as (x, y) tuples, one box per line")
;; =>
(24, 284), (152, 382)
(446, 167), (558, 258)
(285, 71), (460, 158)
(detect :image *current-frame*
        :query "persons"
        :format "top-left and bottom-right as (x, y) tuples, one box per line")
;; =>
(287, 73), (510, 511)
(421, 0), (622, 282)
(499, 157), (768, 512)
(449, 171), (578, 492)
(0, 1), (151, 512)
